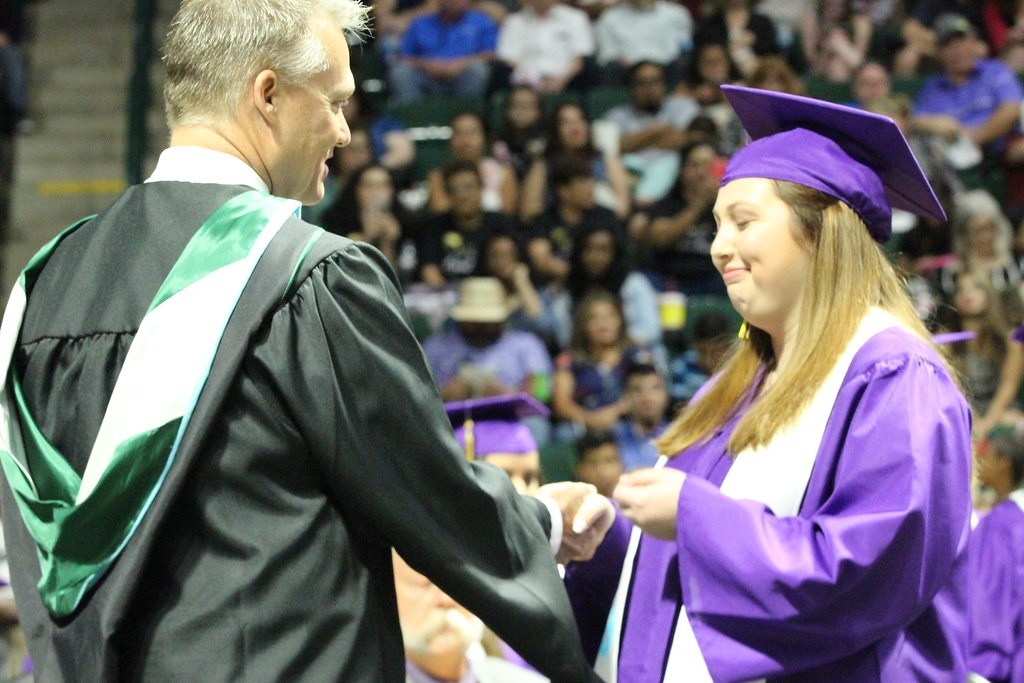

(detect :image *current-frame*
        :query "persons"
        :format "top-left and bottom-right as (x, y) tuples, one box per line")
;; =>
(971, 423), (1024, 683)
(389, 546), (550, 683)
(445, 394), (550, 671)
(0, 0), (602, 683)
(571, 84), (973, 683)
(310, 1), (1024, 524)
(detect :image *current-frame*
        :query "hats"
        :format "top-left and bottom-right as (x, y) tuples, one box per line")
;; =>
(442, 391), (551, 462)
(720, 83), (948, 241)
(447, 277), (509, 324)
(933, 13), (971, 46)
